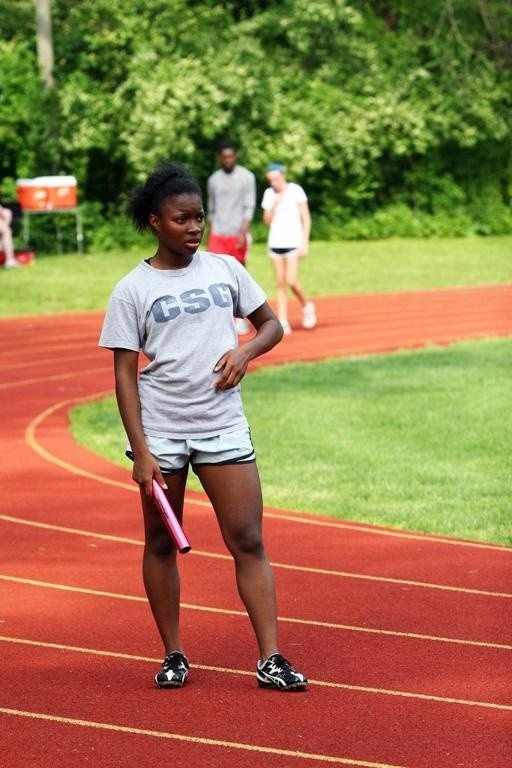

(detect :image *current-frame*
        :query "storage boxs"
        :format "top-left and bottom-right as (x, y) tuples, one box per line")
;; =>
(17, 175), (77, 209)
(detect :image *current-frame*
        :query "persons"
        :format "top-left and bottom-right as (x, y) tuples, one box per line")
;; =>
(208, 147), (256, 334)
(261, 163), (316, 337)
(0, 207), (21, 267)
(97, 163), (307, 691)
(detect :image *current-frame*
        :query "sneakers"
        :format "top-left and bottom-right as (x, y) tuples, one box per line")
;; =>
(155, 652), (190, 687)
(234, 302), (317, 334)
(257, 654), (308, 691)
